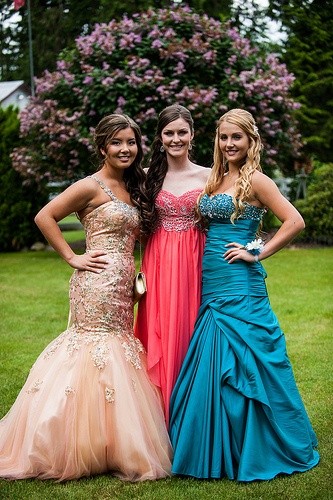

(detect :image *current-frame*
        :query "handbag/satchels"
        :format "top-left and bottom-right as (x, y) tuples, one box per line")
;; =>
(132, 271), (147, 305)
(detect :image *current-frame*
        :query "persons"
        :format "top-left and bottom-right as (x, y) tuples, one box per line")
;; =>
(141, 105), (225, 451)
(34, 113), (148, 469)
(197, 107), (307, 469)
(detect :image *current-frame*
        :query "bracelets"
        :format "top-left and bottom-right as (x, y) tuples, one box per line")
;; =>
(245, 239), (264, 264)
